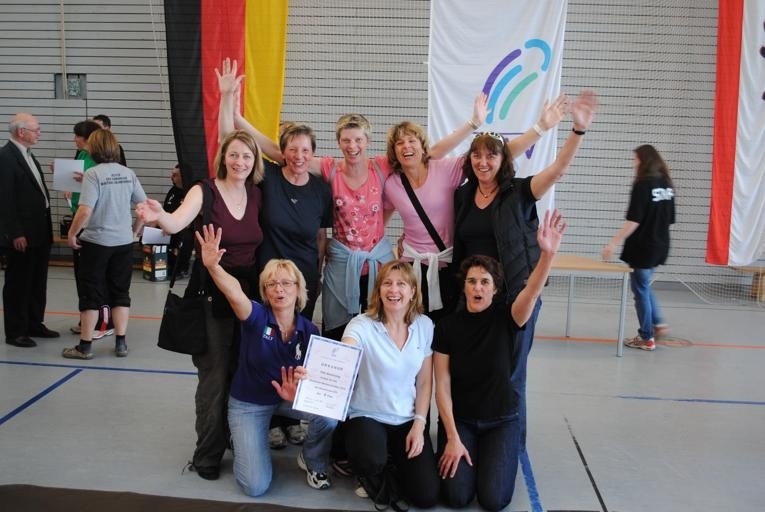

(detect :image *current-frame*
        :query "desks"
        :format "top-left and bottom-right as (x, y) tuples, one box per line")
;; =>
(393, 247), (634, 358)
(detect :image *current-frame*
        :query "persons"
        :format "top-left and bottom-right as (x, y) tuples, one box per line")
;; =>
(0, 113), (60, 347)
(50, 115), (147, 359)
(138, 56), (597, 481)
(603, 145), (676, 350)
(195, 209), (568, 512)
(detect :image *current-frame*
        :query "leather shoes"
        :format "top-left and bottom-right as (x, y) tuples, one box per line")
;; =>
(6, 335), (36, 347)
(28, 324), (59, 338)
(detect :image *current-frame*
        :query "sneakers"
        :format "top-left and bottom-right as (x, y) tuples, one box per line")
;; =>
(177, 270), (188, 280)
(197, 466), (219, 480)
(115, 343), (128, 357)
(655, 324), (668, 335)
(286, 424), (308, 444)
(624, 335), (656, 351)
(62, 345), (94, 359)
(92, 328), (114, 339)
(71, 325), (81, 334)
(352, 474), (368, 498)
(269, 427), (287, 449)
(297, 448), (332, 489)
(332, 456), (352, 480)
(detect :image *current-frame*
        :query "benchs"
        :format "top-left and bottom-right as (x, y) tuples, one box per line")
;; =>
(730, 267), (765, 303)
(47, 236), (161, 269)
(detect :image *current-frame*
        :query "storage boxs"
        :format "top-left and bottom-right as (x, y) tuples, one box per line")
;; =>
(141, 244), (167, 282)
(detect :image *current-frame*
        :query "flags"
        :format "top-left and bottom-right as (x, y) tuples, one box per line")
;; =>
(162, 2), (289, 193)
(426, 0), (569, 232)
(94, 1), (705, 271)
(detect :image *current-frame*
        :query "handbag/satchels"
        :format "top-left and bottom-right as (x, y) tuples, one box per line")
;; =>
(359, 460), (402, 503)
(157, 290), (206, 354)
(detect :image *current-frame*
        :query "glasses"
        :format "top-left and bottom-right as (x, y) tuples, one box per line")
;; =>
(265, 280), (299, 287)
(475, 131), (504, 145)
